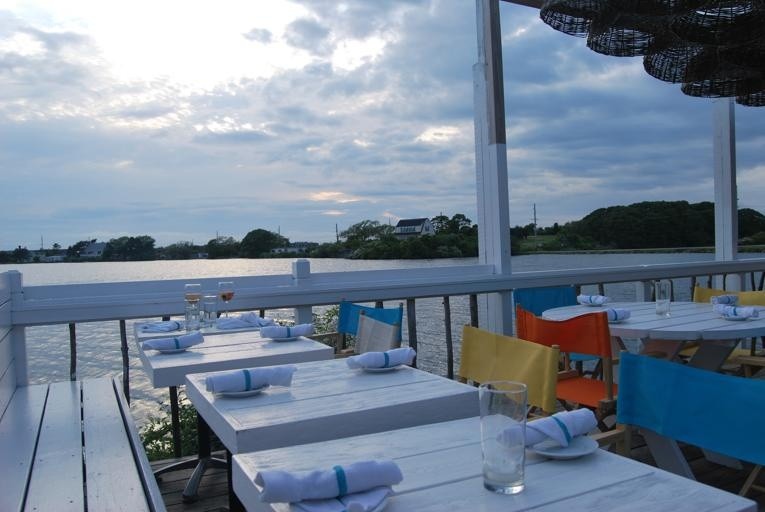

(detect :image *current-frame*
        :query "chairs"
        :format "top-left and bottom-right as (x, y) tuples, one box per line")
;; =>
(461, 323), (558, 418)
(736, 336), (765, 380)
(589, 351), (765, 471)
(678, 282), (765, 375)
(513, 285), (603, 363)
(516, 305), (620, 427)
(337, 297), (402, 354)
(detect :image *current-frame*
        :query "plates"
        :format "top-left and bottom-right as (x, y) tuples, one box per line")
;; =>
(606, 317), (632, 324)
(221, 387), (267, 397)
(159, 349), (185, 353)
(581, 302), (601, 307)
(289, 495), (387, 512)
(273, 336), (297, 342)
(526, 434), (599, 461)
(720, 315), (748, 320)
(363, 368), (393, 373)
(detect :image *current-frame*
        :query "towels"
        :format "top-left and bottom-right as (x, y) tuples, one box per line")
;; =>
(217, 312), (275, 328)
(496, 408), (597, 450)
(140, 321), (183, 333)
(347, 348), (416, 369)
(577, 295), (610, 306)
(712, 295), (739, 306)
(715, 306), (759, 317)
(206, 366), (293, 394)
(142, 331), (203, 351)
(260, 324), (313, 338)
(602, 308), (631, 321)
(254, 461), (403, 512)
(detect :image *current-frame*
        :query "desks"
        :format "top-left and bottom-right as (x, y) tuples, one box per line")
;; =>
(542, 300), (764, 369)
(133, 318), (335, 504)
(230, 413), (759, 512)
(183, 359), (480, 455)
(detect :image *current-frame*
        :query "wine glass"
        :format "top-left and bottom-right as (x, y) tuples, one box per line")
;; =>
(217, 281), (233, 318)
(183, 283), (200, 307)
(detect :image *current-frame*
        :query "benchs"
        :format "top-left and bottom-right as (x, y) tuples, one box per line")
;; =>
(2, 274), (165, 512)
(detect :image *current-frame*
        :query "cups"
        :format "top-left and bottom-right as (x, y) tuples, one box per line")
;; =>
(477, 380), (528, 496)
(183, 298), (200, 331)
(654, 282), (671, 315)
(202, 296), (216, 323)
